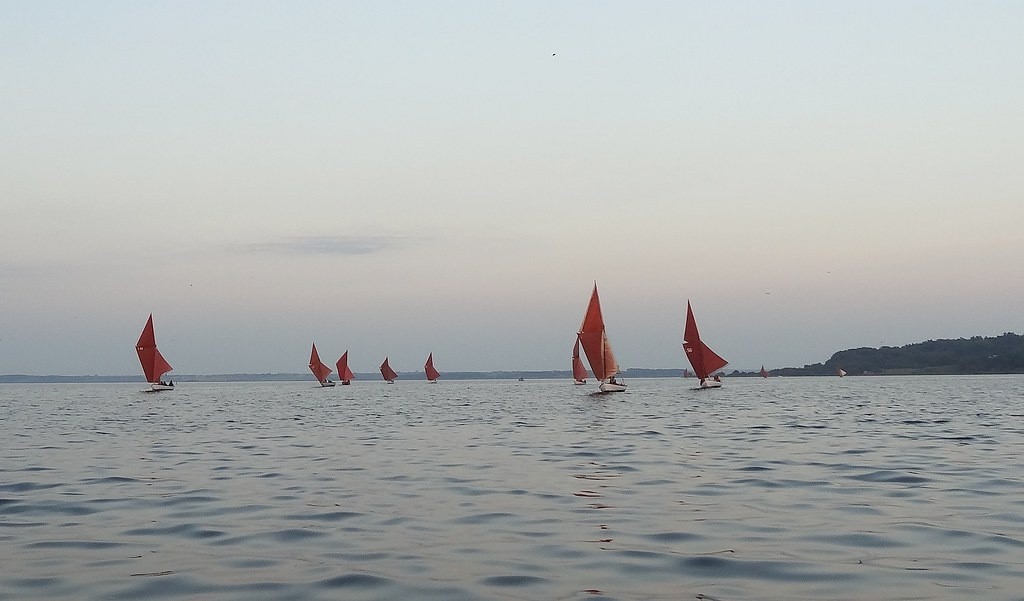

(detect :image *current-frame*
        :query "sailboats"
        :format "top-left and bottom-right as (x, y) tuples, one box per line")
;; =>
(423, 352), (443, 384)
(134, 313), (180, 389)
(575, 280), (634, 395)
(571, 336), (589, 384)
(760, 365), (768, 378)
(308, 342), (337, 386)
(334, 350), (355, 385)
(379, 357), (399, 383)
(681, 299), (730, 391)
(837, 368), (849, 378)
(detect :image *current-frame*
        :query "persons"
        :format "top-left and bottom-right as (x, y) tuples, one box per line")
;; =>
(161, 380), (173, 386)
(328, 380), (332, 383)
(610, 376), (616, 384)
(700, 378), (705, 386)
(713, 374), (721, 382)
(347, 379), (350, 382)
